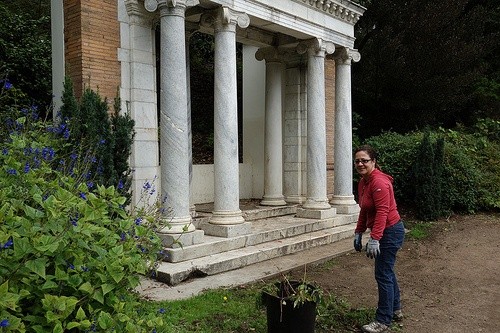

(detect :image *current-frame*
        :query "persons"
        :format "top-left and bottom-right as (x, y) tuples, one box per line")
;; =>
(353, 145), (405, 333)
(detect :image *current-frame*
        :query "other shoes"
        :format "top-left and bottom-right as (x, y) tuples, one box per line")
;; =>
(362, 308), (404, 333)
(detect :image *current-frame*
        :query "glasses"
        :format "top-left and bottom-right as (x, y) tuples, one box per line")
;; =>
(354, 157), (373, 164)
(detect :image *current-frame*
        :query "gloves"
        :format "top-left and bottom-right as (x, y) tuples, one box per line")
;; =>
(353, 233), (381, 258)
